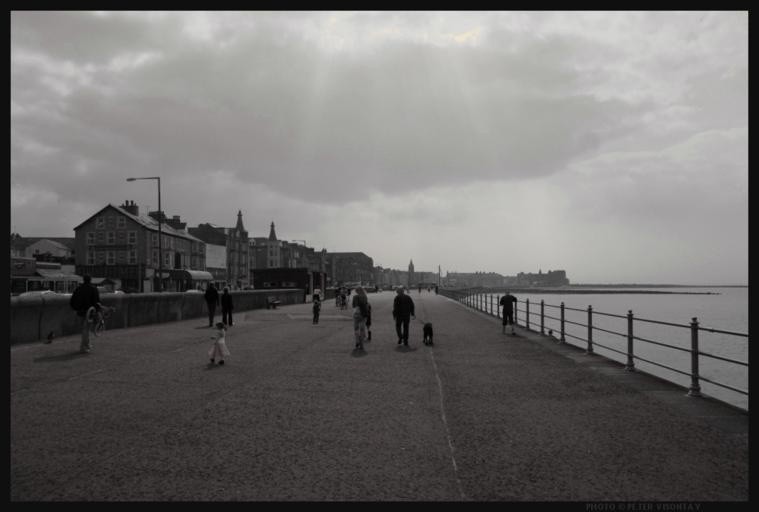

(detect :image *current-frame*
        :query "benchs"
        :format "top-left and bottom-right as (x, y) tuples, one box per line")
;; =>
(267, 296), (283, 309)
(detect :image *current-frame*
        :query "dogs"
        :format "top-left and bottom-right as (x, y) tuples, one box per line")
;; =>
(422, 322), (434, 346)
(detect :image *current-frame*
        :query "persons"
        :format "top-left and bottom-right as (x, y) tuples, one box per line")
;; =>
(70, 273), (102, 354)
(334, 285), (371, 350)
(392, 285), (415, 346)
(313, 285), (323, 306)
(312, 300), (321, 324)
(209, 322), (230, 366)
(203, 280), (220, 327)
(221, 287), (235, 327)
(418, 284), (422, 293)
(500, 291), (517, 336)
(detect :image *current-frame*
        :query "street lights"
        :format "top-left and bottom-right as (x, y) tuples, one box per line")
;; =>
(126, 177), (162, 292)
(293, 239), (306, 264)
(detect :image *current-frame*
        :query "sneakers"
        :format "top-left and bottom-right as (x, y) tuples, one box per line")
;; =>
(80, 344), (93, 353)
(209, 358), (224, 364)
